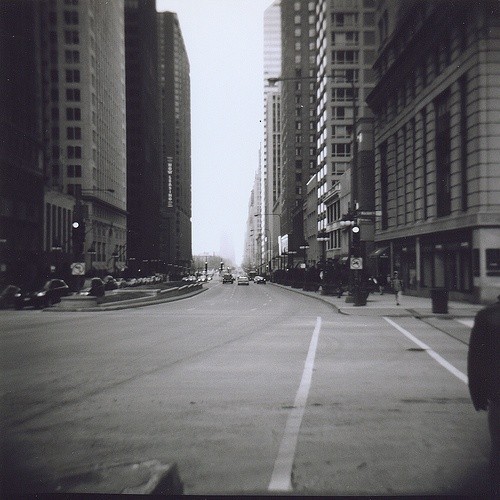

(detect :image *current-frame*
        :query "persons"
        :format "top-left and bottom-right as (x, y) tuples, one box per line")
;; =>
(391, 271), (402, 305)
(374, 273), (384, 295)
(467, 294), (500, 494)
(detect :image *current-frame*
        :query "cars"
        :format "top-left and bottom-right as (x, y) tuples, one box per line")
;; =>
(223, 271), (266, 286)
(13, 277), (69, 310)
(101, 266), (214, 292)
(67, 277), (104, 297)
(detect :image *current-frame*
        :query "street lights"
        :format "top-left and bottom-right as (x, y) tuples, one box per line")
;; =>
(72, 187), (116, 294)
(275, 245), (298, 288)
(316, 232), (331, 295)
(339, 206), (361, 303)
(254, 213), (283, 270)
(299, 240), (310, 291)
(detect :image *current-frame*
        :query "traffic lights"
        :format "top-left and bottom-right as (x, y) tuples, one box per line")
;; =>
(72, 219), (82, 242)
(352, 226), (360, 247)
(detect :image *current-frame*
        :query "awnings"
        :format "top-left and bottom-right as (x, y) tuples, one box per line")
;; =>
(369, 246), (389, 258)
